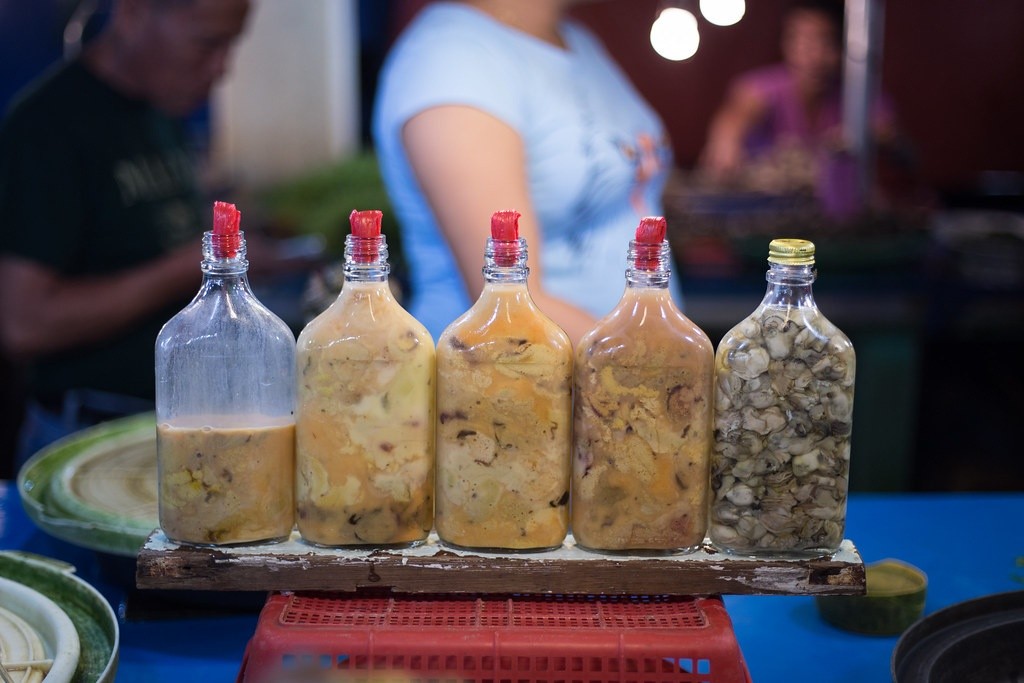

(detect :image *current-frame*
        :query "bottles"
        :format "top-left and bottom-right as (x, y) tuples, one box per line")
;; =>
(575, 236), (717, 554)
(715, 238), (857, 560)
(296, 234), (437, 550)
(155, 230), (297, 551)
(438, 238), (574, 553)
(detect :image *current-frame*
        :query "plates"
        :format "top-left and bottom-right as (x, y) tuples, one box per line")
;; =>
(1, 549), (120, 683)
(16, 407), (155, 559)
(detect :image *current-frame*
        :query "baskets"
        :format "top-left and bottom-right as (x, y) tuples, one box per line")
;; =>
(238, 591), (751, 683)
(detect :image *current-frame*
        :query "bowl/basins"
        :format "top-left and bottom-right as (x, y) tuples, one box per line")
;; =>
(889, 590), (1024, 683)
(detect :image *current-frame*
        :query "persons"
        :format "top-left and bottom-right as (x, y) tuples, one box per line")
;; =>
(374, 0), (688, 346)
(697, 0), (1024, 494)
(0, 0), (327, 490)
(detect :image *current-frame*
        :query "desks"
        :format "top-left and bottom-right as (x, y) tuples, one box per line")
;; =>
(0, 478), (1024, 683)
